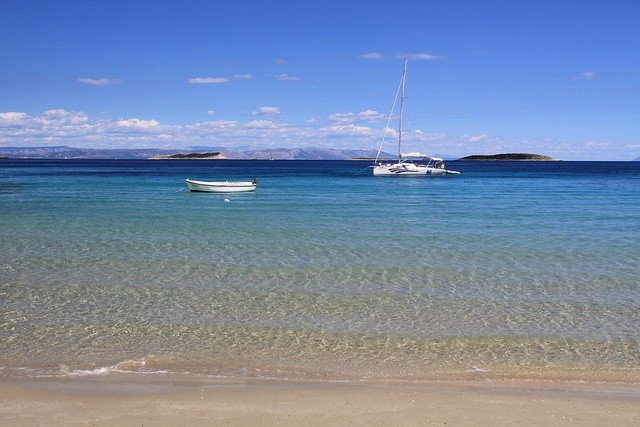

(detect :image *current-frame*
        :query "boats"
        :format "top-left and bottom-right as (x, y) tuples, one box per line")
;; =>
(185, 179), (257, 192)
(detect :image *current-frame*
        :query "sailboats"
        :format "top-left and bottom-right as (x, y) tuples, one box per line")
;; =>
(369, 57), (461, 176)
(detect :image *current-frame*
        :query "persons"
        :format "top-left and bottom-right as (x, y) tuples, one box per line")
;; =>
(415, 161), (421, 166)
(440, 162), (446, 169)
